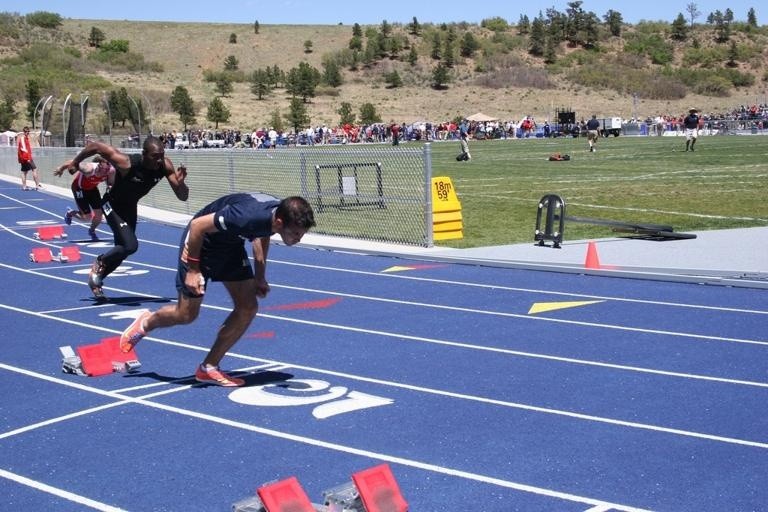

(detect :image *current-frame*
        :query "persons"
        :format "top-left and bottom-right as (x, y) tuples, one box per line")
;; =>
(120, 193), (315, 388)
(121, 120), (454, 148)
(684, 108), (699, 153)
(623, 103), (768, 136)
(68, 137), (189, 296)
(468, 115), (586, 140)
(586, 114), (601, 152)
(459, 120), (471, 160)
(16, 126), (43, 192)
(53, 154), (116, 240)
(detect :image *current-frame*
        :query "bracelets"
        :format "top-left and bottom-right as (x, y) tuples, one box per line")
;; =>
(187, 256), (201, 263)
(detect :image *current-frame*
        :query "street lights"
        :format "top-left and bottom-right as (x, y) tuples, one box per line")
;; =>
(632, 91), (638, 120)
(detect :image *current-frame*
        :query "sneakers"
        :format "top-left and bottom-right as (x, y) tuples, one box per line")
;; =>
(195, 364), (246, 387)
(119, 310), (153, 353)
(22, 183), (45, 191)
(88, 253), (107, 302)
(63, 206), (72, 225)
(88, 228), (99, 242)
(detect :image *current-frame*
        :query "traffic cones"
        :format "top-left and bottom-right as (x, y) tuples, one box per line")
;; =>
(583, 238), (601, 269)
